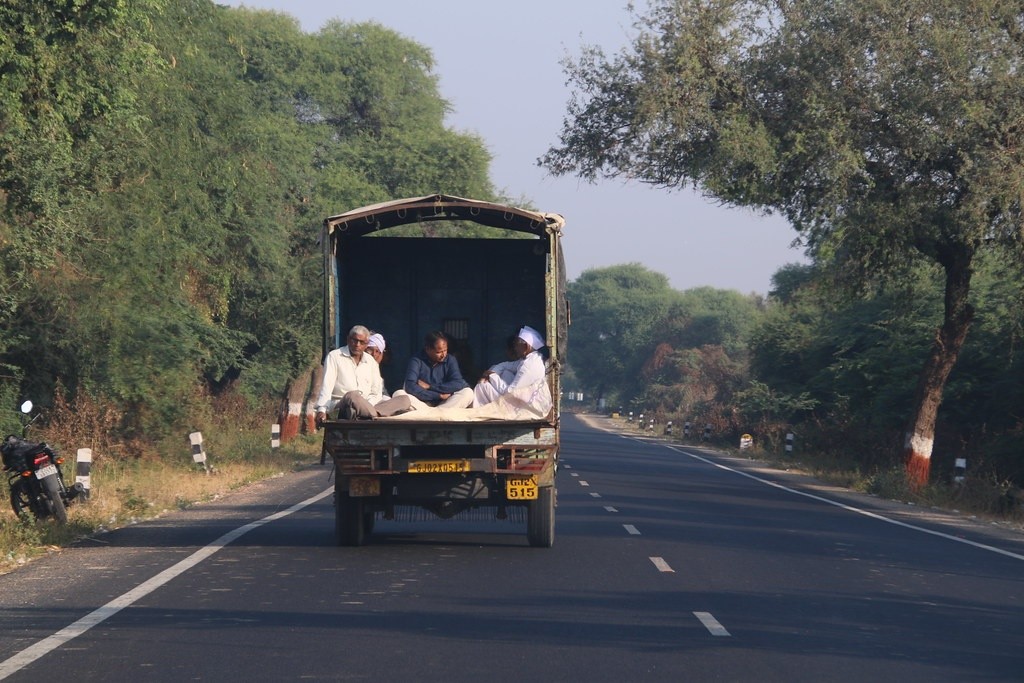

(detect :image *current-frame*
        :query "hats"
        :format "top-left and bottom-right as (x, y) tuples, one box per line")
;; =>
(367, 333), (386, 353)
(519, 326), (545, 350)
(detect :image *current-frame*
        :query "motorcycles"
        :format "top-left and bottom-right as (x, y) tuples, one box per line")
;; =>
(0, 400), (84, 530)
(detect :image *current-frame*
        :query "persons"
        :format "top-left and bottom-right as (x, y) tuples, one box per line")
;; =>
(392, 332), (474, 411)
(470, 324), (545, 408)
(313, 324), (411, 431)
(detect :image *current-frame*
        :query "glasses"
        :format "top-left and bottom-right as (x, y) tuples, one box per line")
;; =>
(351, 337), (368, 345)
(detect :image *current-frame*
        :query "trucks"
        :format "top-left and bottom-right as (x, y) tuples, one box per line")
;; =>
(320, 193), (566, 548)
